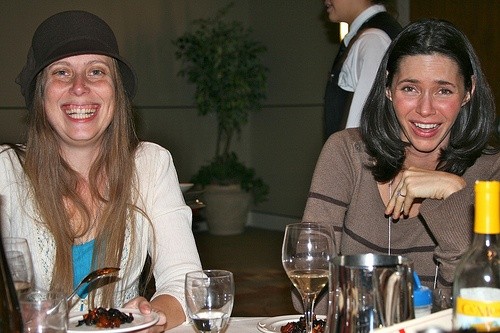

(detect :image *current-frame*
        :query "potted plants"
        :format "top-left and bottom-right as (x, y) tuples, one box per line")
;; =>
(169, 3), (272, 236)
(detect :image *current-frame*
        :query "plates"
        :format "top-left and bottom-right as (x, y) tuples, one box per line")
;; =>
(257, 313), (327, 333)
(46, 308), (158, 333)
(179, 183), (194, 193)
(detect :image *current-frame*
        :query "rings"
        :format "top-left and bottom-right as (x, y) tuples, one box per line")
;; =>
(396, 190), (406, 197)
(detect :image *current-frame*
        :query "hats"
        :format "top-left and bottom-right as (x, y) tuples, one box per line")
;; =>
(15, 9), (138, 109)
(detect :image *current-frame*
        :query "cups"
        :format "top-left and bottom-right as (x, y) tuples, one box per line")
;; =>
(1, 237), (35, 323)
(19, 290), (67, 333)
(185, 269), (234, 333)
(325, 252), (413, 333)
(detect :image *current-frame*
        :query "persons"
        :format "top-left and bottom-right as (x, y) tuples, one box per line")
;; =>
(291, 18), (500, 315)
(320, 0), (404, 144)
(0, 12), (212, 332)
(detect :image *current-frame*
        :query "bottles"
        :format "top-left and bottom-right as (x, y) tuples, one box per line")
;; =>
(452, 179), (500, 333)
(411, 286), (433, 318)
(0, 232), (24, 333)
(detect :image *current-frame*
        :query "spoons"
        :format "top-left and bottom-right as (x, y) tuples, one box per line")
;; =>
(47, 267), (120, 314)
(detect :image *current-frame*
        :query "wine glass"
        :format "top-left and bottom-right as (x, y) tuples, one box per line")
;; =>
(281, 221), (336, 333)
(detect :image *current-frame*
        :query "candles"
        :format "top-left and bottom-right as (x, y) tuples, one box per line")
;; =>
(474, 180), (500, 237)
